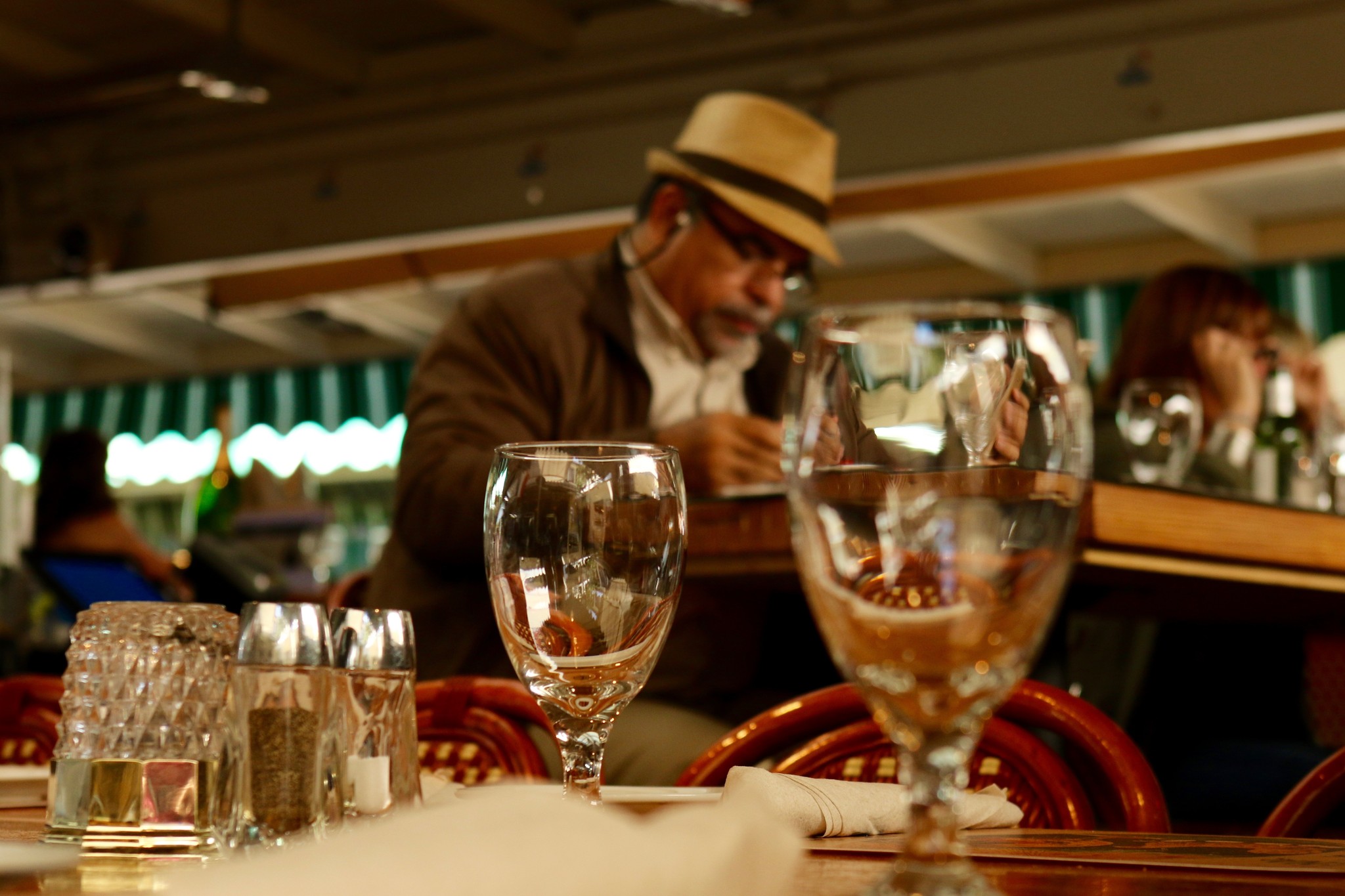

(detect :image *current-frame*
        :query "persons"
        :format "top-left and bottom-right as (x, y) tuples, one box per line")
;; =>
(360, 90), (845, 788)
(1089, 259), (1345, 485)
(33, 426), (195, 605)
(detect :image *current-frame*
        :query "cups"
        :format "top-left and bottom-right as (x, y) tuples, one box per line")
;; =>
(1121, 379), (1204, 488)
(35, 596), (256, 863)
(1204, 422), (1345, 516)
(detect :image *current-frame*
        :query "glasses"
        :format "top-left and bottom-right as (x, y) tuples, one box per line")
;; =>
(688, 191), (818, 292)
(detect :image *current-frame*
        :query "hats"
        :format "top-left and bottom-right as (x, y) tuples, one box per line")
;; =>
(646, 90), (847, 268)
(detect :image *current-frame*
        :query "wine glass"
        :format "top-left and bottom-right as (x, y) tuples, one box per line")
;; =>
(776, 297), (1092, 896)
(482, 439), (689, 810)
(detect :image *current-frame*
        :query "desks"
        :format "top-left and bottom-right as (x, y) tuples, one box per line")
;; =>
(671, 479), (1343, 825)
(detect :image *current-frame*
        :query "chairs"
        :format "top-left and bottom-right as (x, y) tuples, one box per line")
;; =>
(420, 673), (606, 794)
(669, 683), (1166, 831)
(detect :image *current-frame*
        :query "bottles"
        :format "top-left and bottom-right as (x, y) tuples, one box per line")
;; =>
(1252, 367), (1308, 507)
(234, 600), (343, 848)
(330, 606), (424, 827)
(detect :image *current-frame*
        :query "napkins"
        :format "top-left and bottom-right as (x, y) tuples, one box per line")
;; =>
(716, 765), (1025, 837)
